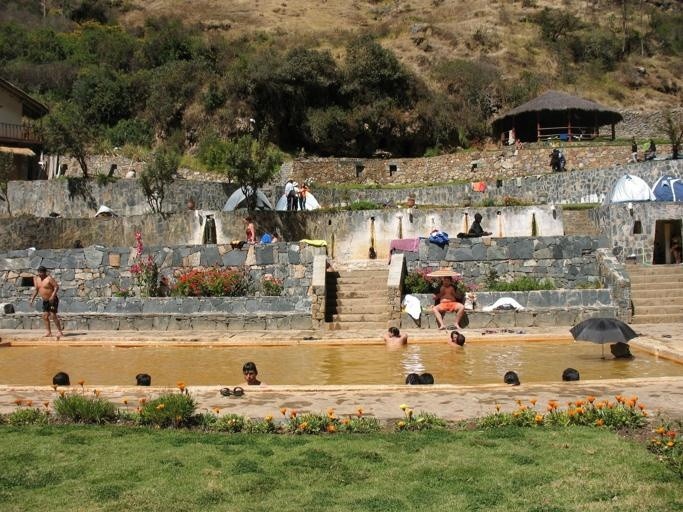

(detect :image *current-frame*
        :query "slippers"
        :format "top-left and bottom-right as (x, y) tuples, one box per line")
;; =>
(220, 388), (231, 396)
(234, 387), (244, 397)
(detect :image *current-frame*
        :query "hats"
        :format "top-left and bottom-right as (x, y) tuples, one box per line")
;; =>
(286, 178), (293, 182)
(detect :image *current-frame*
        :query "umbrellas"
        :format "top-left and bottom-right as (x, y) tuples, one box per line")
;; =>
(568, 317), (637, 359)
(425, 268), (462, 287)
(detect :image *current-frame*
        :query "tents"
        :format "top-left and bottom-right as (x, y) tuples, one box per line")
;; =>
(650, 173), (683, 202)
(274, 189), (322, 211)
(221, 185), (273, 211)
(599, 174), (656, 205)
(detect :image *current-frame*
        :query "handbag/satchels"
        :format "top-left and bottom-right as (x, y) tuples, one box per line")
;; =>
(291, 190), (295, 196)
(428, 229), (449, 245)
(299, 191), (305, 200)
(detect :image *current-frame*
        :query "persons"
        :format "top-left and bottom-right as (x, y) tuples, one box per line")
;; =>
(405, 372), (419, 385)
(449, 330), (458, 343)
(52, 371), (70, 385)
(383, 326), (407, 347)
(669, 230), (682, 264)
(237, 361), (268, 385)
(417, 372), (434, 384)
(509, 126), (515, 139)
(29, 266), (64, 337)
(431, 275), (464, 330)
(291, 181), (304, 211)
(503, 371), (520, 385)
(554, 150), (567, 171)
(630, 141), (637, 163)
(548, 149), (557, 172)
(133, 372), (151, 386)
(465, 212), (493, 238)
(270, 231), (278, 243)
(284, 177), (294, 210)
(54, 163), (67, 178)
(124, 170), (136, 179)
(515, 139), (524, 150)
(447, 334), (466, 348)
(244, 216), (256, 244)
(561, 367), (580, 381)
(298, 182), (310, 211)
(646, 139), (656, 159)
(610, 341), (632, 358)
